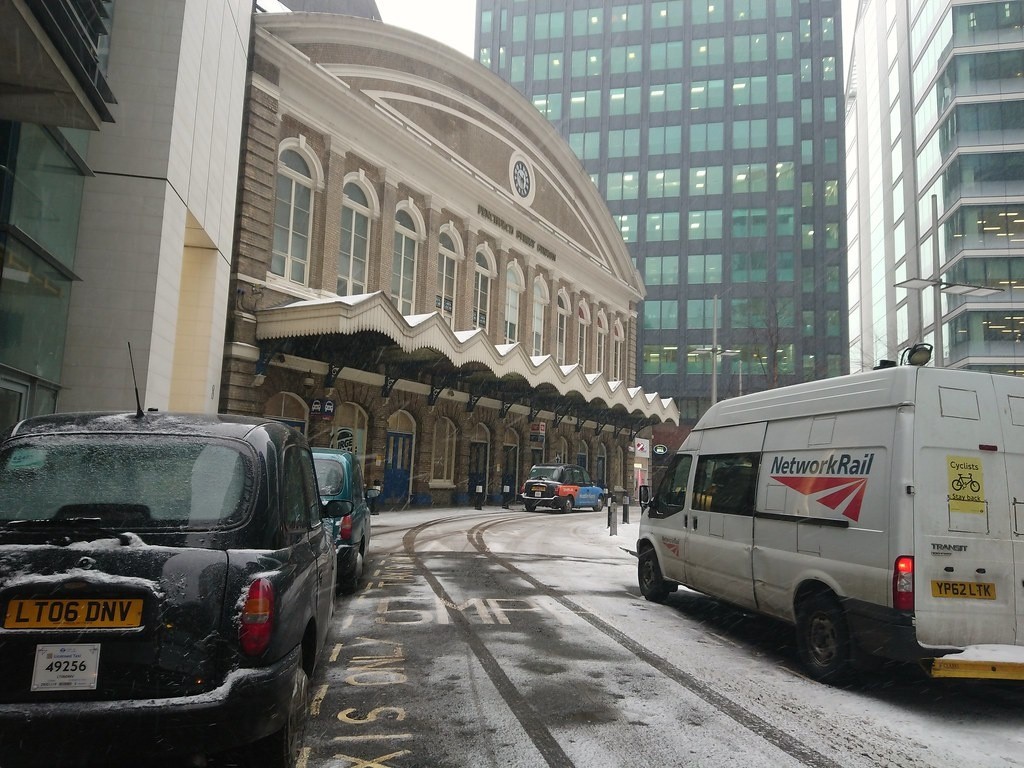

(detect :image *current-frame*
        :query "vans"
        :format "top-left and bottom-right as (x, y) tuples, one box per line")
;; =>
(636, 363), (1024, 681)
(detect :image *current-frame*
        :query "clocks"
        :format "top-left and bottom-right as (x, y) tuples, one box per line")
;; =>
(509, 149), (536, 206)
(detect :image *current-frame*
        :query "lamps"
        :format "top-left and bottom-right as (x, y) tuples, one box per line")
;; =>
(303, 369), (314, 390)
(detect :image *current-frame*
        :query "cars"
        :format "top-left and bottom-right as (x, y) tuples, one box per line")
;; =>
(0, 409), (353, 767)
(520, 462), (605, 514)
(310, 445), (368, 592)
